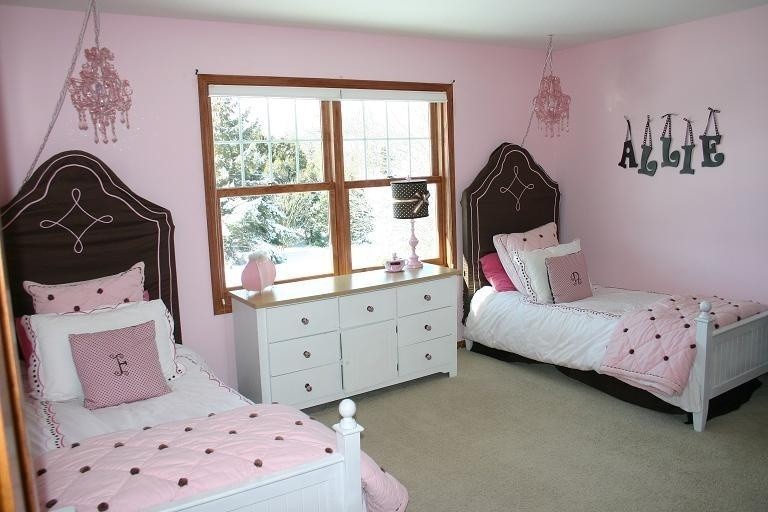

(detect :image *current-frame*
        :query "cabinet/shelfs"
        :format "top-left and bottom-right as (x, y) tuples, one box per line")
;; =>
(229, 258), (458, 407)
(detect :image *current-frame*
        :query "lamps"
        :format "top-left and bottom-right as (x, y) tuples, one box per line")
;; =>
(390, 179), (430, 269)
(520, 33), (572, 151)
(18, 1), (134, 189)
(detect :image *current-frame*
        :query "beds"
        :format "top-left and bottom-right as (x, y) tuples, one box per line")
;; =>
(460, 142), (768, 431)
(0, 150), (365, 512)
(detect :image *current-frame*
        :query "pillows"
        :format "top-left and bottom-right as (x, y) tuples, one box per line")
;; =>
(480, 222), (593, 305)
(15, 262), (185, 412)
